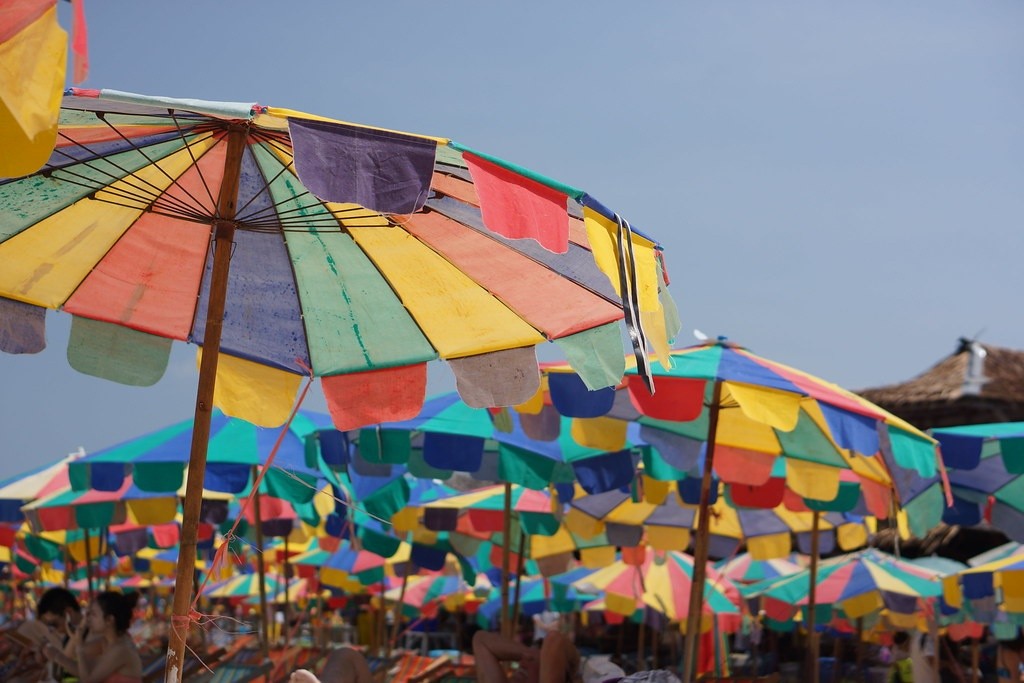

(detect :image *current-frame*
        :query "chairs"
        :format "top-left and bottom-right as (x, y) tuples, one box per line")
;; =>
(0, 585), (1021, 683)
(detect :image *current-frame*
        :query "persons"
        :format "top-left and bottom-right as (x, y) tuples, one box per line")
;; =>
(37, 587), (89, 683)
(65, 591), (142, 683)
(887, 632), (913, 683)
(289, 630), (581, 683)
(997, 641), (1024, 683)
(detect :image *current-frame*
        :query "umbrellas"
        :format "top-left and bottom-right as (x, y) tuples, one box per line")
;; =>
(0, 0), (69, 179)
(0, 89), (681, 683)
(0, 336), (1024, 683)
